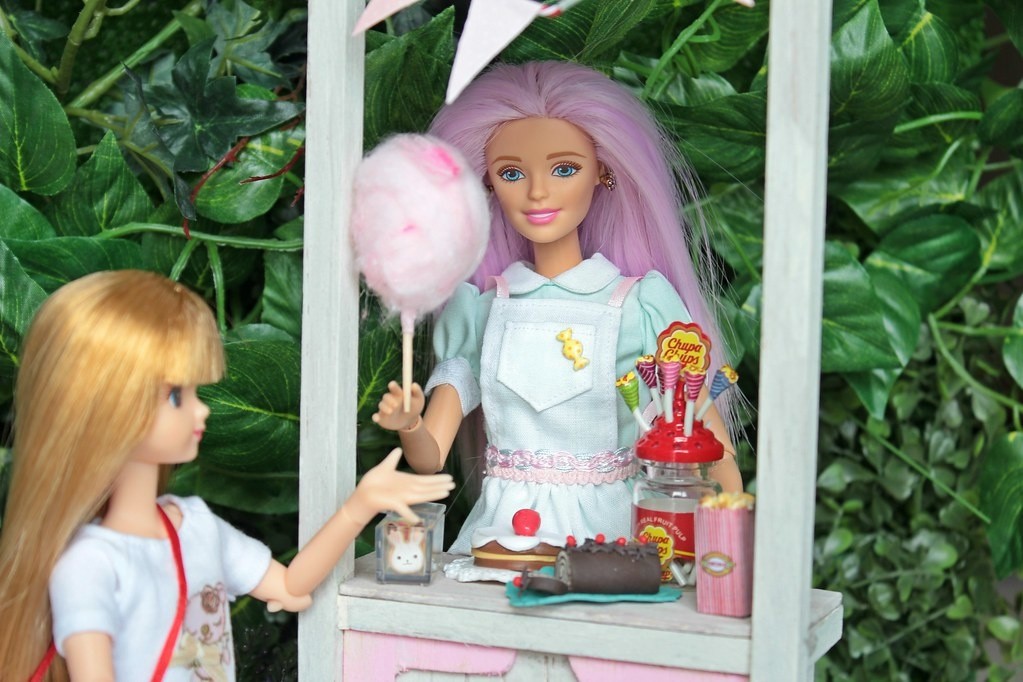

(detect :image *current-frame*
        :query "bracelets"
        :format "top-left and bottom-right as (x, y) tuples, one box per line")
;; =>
(398, 414), (422, 433)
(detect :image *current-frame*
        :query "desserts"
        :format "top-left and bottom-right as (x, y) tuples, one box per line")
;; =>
(514, 535), (663, 596)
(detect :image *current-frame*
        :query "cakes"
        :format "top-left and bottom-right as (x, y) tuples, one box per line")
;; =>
(470, 508), (575, 575)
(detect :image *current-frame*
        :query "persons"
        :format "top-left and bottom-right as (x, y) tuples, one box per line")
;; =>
(0, 269), (456, 682)
(371, 61), (743, 558)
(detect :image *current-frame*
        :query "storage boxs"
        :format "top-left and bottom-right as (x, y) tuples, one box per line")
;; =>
(376, 504), (446, 589)
